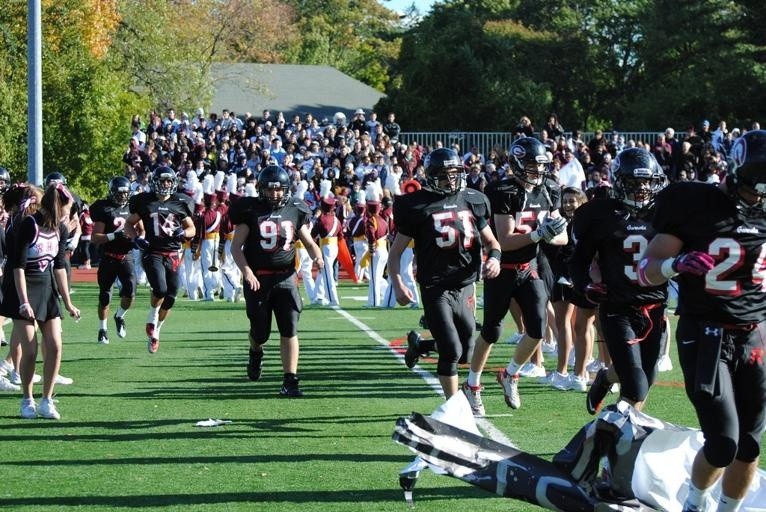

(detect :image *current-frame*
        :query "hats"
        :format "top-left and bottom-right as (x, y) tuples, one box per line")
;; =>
(183, 188), (382, 214)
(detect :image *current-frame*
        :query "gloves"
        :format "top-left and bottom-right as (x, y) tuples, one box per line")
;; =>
(531, 212), (565, 243)
(673, 250), (713, 275)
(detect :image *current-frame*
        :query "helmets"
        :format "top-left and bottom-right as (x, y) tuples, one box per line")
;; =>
(1, 168), (67, 187)
(257, 168), (291, 200)
(611, 148), (666, 206)
(425, 148), (464, 193)
(151, 166), (177, 192)
(727, 129), (766, 210)
(509, 138), (550, 188)
(108, 177), (133, 195)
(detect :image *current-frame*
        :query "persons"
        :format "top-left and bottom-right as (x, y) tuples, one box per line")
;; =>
(636, 130), (766, 512)
(2, 105), (765, 428)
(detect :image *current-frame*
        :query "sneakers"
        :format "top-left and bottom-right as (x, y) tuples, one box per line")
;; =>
(99, 331), (108, 343)
(76, 263), (93, 272)
(21, 397), (59, 420)
(181, 291), (244, 302)
(303, 296), (421, 308)
(586, 366), (612, 415)
(247, 348), (262, 381)
(1, 358), (74, 393)
(496, 371), (521, 409)
(406, 332), (420, 367)
(147, 311), (160, 353)
(462, 381), (484, 415)
(507, 333), (606, 391)
(113, 314), (127, 338)
(281, 375), (302, 396)
